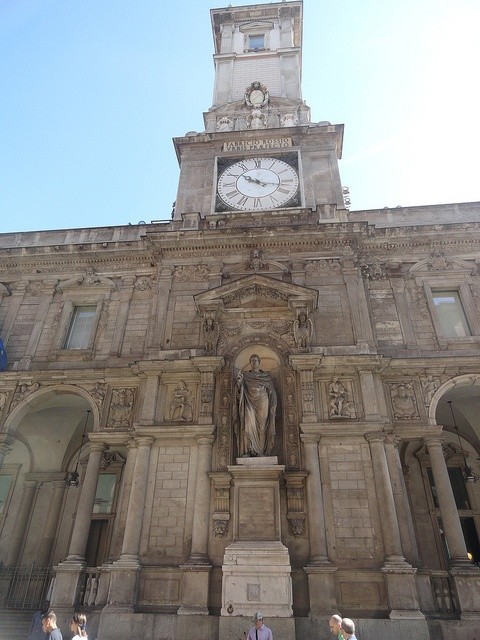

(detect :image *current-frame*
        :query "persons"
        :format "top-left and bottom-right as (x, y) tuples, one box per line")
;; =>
(230, 354), (279, 456)
(329, 614), (345, 640)
(328, 375), (346, 416)
(69, 613), (88, 640)
(41, 610), (63, 640)
(29, 601), (50, 640)
(107, 392), (132, 427)
(170, 380), (189, 420)
(242, 611), (273, 640)
(340, 618), (357, 640)
(392, 385), (420, 420)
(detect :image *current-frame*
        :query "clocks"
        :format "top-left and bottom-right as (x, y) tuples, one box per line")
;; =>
(216, 157), (301, 212)
